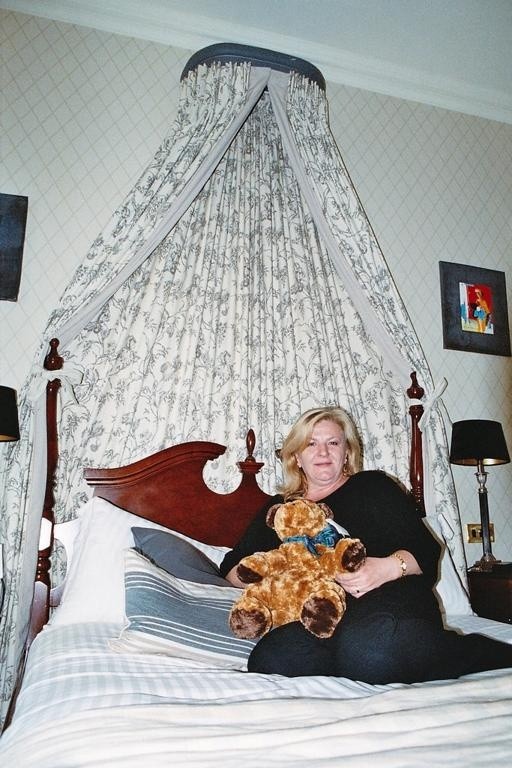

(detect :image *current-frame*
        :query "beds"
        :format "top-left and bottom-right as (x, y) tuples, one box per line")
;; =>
(1, 338), (510, 768)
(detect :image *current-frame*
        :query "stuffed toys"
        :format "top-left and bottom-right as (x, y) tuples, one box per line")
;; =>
(226, 498), (370, 640)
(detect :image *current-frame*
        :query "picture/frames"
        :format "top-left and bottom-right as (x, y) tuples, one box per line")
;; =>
(438, 259), (511, 358)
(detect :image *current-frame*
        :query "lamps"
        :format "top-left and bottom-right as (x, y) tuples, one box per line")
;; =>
(448, 419), (511, 576)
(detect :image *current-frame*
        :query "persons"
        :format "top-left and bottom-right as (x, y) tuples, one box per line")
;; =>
(472, 287), (490, 332)
(219, 405), (511, 686)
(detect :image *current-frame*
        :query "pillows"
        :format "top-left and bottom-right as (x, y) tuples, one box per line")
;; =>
(42, 495), (261, 672)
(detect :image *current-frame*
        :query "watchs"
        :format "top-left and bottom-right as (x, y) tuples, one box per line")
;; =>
(389, 552), (409, 579)
